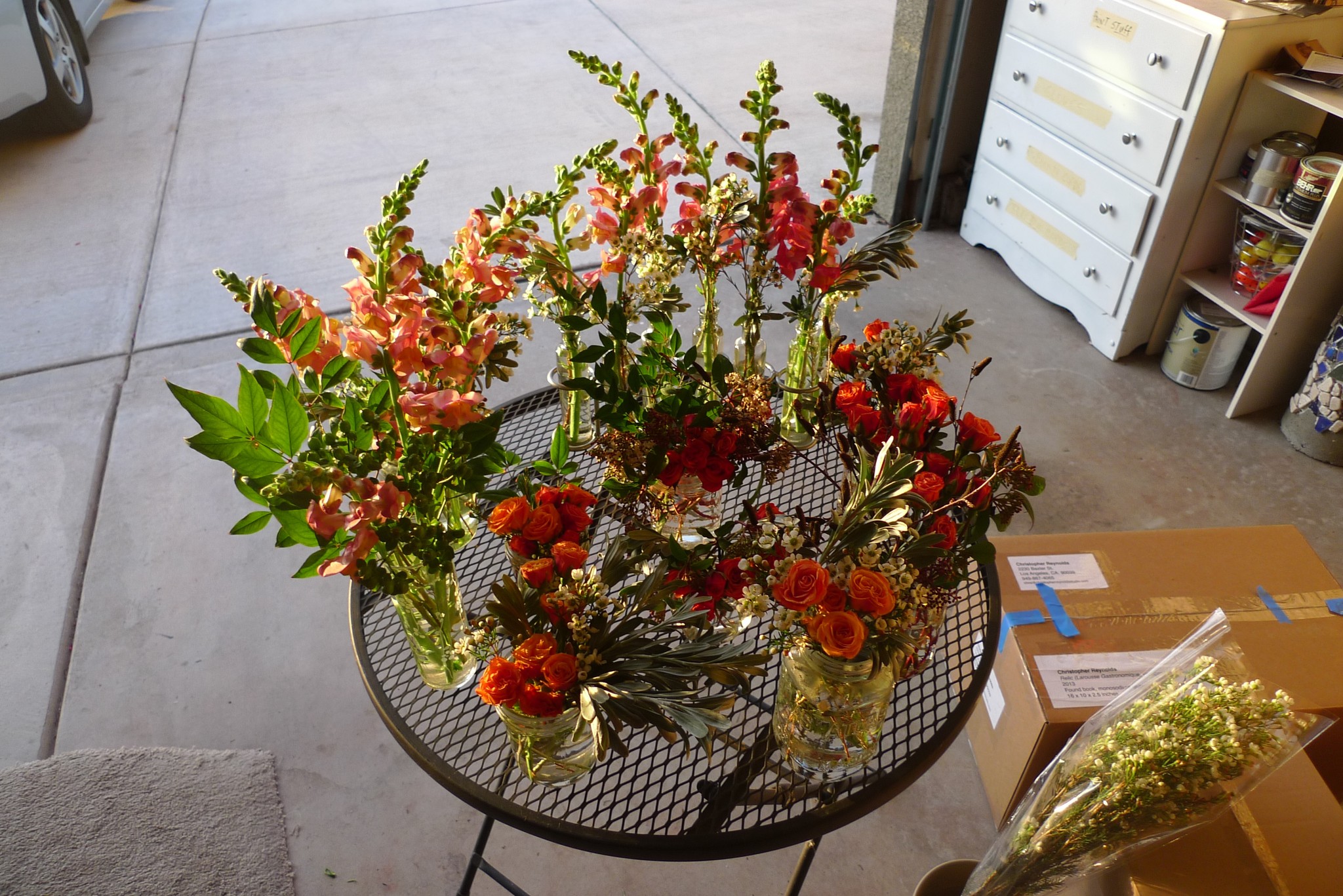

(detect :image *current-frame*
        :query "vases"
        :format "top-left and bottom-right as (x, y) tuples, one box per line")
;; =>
(913, 859), (983, 896)
(380, 273), (956, 784)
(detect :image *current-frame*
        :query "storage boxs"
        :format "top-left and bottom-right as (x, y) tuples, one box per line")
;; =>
(1076, 724), (1342, 896)
(945, 523), (1340, 834)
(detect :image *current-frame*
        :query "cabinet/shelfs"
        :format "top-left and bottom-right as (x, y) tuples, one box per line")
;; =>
(958, 0), (1343, 420)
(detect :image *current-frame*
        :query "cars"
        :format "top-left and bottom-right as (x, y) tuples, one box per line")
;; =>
(0, 0), (114, 132)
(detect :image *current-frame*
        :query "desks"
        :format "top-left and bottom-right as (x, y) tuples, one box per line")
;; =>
(344, 381), (1001, 895)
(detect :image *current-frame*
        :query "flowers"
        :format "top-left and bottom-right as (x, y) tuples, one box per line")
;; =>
(168, 41), (1044, 694)
(962, 605), (1337, 895)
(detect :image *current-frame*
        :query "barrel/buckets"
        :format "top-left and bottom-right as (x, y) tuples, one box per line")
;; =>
(1160, 292), (1254, 390)
(1241, 130), (1343, 229)
